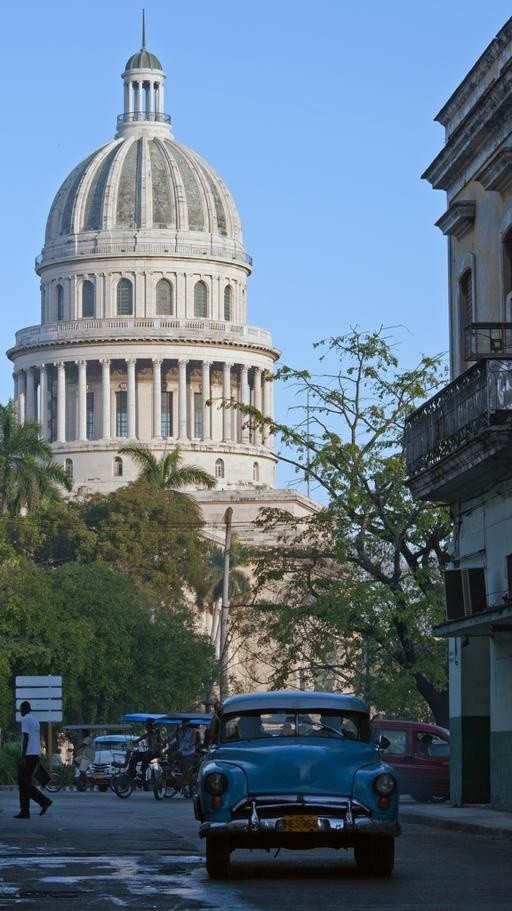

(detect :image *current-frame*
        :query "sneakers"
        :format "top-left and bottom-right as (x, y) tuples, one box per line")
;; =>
(11, 800), (54, 820)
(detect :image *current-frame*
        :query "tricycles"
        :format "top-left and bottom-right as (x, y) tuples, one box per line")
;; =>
(109, 739), (209, 800)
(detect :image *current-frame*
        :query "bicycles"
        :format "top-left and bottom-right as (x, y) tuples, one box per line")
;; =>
(43, 758), (89, 794)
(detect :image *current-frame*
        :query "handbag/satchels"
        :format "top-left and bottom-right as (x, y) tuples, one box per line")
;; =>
(32, 763), (51, 789)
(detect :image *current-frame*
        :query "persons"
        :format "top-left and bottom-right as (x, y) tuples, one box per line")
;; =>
(417, 734), (434, 757)
(310, 715), (356, 738)
(13, 700), (54, 819)
(41, 718), (201, 792)
(237, 715), (272, 738)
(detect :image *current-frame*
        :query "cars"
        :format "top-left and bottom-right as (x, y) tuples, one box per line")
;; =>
(191, 688), (402, 881)
(82, 732), (157, 793)
(366, 717), (454, 804)
